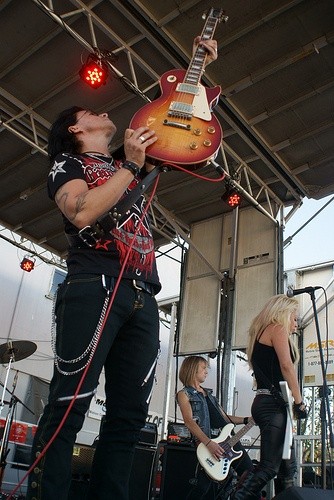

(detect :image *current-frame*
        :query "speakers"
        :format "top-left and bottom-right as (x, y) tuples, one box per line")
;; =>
(128, 446), (158, 500)
(100, 415), (157, 447)
(159, 444), (199, 500)
(271, 487), (334, 500)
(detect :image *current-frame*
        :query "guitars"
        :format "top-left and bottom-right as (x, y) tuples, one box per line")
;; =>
(128, 6), (229, 176)
(278, 381), (298, 460)
(196, 417), (256, 481)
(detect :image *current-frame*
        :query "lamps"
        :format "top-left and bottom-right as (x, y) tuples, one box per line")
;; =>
(220, 185), (243, 210)
(79, 53), (109, 90)
(21, 254), (36, 272)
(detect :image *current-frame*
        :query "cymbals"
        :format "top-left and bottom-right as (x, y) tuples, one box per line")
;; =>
(0, 340), (37, 364)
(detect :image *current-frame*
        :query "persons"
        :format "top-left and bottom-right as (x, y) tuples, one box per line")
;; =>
(175, 356), (253, 499)
(26, 36), (219, 500)
(229, 295), (307, 500)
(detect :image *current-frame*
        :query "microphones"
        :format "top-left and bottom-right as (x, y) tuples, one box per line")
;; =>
(287, 286), (322, 297)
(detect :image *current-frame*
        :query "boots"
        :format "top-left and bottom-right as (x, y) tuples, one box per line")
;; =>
(232, 468), (277, 500)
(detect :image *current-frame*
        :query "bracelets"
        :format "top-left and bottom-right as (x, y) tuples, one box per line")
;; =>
(122, 160), (140, 178)
(294, 402), (304, 406)
(205, 440), (211, 446)
(244, 417), (248, 424)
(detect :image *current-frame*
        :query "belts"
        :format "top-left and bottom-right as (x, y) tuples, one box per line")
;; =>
(122, 277), (159, 299)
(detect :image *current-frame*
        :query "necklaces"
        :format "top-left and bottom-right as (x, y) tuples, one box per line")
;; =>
(82, 151), (108, 156)
